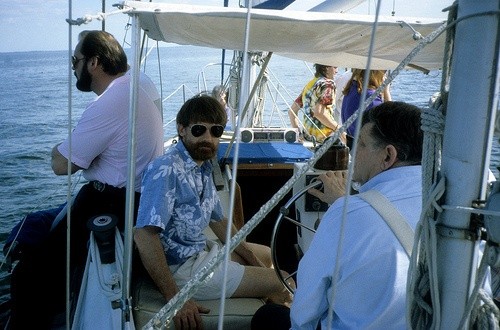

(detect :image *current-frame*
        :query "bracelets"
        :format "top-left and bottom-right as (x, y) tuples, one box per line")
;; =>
(335, 124), (338, 130)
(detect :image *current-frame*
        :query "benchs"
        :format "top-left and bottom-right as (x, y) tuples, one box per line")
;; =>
(131, 280), (265, 330)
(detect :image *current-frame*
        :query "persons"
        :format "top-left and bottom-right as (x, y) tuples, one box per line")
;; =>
(289, 64), (346, 143)
(134, 96), (296, 330)
(341, 68), (392, 156)
(290, 102), (422, 330)
(48, 30), (163, 244)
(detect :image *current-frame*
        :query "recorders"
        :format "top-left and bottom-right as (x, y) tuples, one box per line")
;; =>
(239, 128), (299, 144)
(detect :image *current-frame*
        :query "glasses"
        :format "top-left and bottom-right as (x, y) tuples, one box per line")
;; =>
(71, 55), (96, 70)
(185, 124), (224, 138)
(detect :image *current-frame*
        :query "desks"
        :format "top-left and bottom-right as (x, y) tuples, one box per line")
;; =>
(217, 142), (314, 170)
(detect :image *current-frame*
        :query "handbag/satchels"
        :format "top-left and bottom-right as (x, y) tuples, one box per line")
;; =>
(70, 226), (135, 330)
